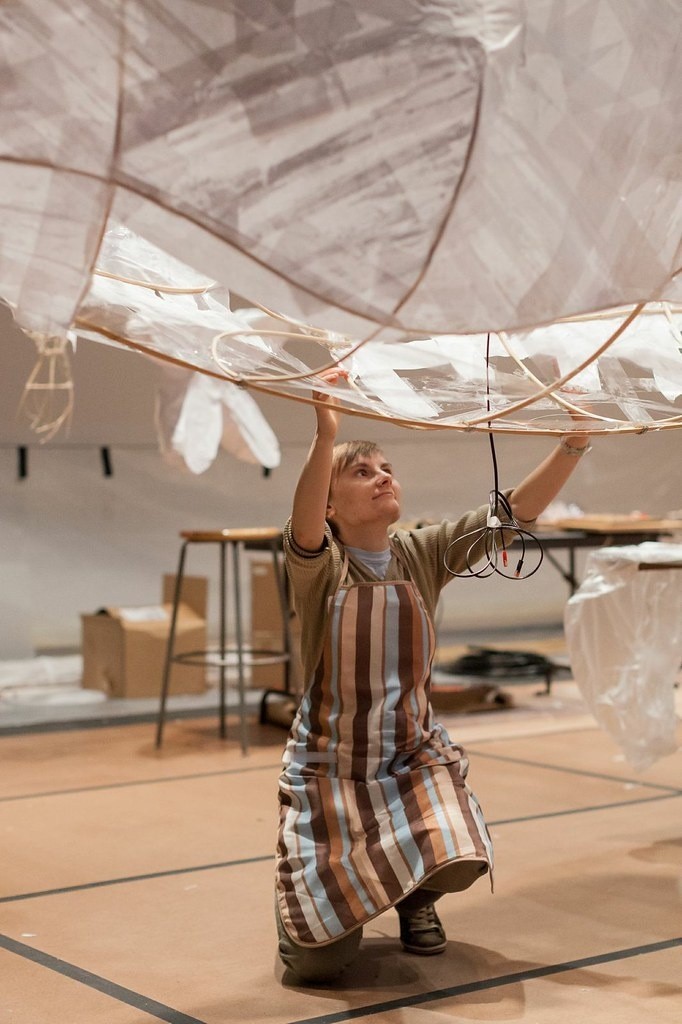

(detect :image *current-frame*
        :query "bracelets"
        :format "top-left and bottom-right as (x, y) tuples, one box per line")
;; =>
(560, 437), (591, 456)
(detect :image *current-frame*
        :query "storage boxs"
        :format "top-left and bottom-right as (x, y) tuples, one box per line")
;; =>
(247, 560), (291, 693)
(77, 573), (207, 699)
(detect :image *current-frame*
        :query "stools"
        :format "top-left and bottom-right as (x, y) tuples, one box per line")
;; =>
(155, 527), (291, 748)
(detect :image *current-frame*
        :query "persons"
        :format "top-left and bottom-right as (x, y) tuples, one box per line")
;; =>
(275, 365), (592, 982)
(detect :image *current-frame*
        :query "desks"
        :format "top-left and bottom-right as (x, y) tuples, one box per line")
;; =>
(249, 527), (672, 681)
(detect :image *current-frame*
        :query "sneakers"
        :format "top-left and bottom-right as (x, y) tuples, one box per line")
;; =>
(396, 903), (450, 954)
